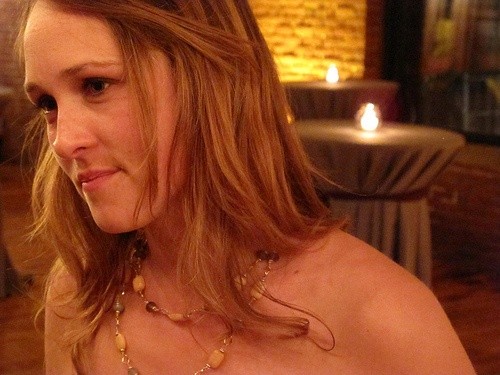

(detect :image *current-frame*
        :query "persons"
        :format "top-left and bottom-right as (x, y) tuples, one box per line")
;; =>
(10, 0), (475, 375)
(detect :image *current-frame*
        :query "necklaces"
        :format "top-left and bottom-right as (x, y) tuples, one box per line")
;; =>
(111, 235), (280, 375)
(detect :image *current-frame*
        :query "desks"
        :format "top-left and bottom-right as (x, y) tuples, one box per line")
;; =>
(283, 79), (466, 292)
